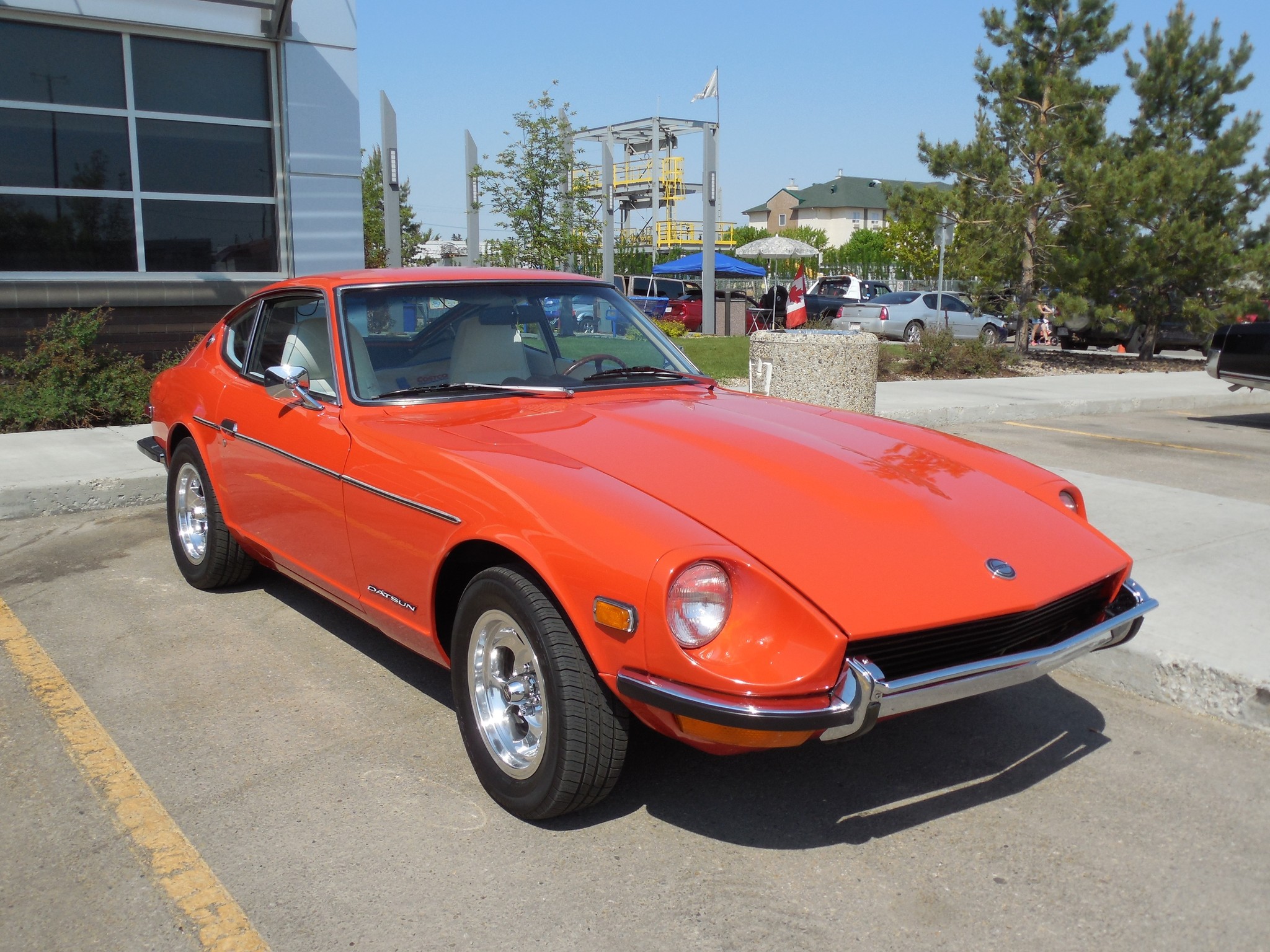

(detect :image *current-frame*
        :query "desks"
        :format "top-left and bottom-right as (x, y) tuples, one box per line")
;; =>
(746, 307), (773, 336)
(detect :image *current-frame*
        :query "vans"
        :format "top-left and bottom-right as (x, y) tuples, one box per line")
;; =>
(542, 273), (702, 335)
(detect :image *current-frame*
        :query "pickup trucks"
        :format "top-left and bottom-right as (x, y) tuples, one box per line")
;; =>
(759, 273), (894, 328)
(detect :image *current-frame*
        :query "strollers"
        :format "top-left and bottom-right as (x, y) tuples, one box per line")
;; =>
(1050, 314), (1061, 346)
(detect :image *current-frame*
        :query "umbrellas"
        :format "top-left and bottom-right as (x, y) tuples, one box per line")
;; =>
(735, 234), (819, 330)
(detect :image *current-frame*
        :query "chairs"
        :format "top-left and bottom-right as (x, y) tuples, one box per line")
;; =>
(284, 316), (382, 406)
(866, 288), (877, 299)
(827, 287), (845, 296)
(448, 312), (529, 387)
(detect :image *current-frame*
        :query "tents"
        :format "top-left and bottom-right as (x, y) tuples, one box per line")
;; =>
(643, 251), (768, 313)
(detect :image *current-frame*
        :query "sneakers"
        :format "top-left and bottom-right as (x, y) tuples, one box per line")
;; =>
(1030, 341), (1039, 346)
(1046, 342), (1050, 346)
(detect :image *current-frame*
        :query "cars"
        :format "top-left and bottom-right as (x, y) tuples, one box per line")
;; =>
(653, 289), (780, 336)
(1053, 287), (1270, 392)
(138, 265), (1161, 821)
(831, 289), (1034, 346)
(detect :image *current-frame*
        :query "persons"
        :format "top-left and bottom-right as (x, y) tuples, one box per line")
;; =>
(1029, 300), (1056, 346)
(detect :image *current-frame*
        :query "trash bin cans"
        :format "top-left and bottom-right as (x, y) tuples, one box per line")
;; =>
(748, 328), (878, 414)
(714, 287), (746, 337)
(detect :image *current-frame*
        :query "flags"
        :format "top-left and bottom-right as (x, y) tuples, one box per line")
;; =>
(786, 260), (808, 329)
(691, 69), (718, 103)
